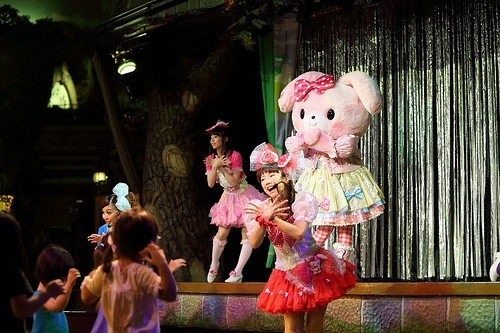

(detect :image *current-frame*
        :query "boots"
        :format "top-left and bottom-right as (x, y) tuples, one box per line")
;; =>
(333, 242), (353, 261)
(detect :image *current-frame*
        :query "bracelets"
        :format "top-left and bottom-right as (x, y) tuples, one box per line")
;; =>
(255, 214), (278, 229)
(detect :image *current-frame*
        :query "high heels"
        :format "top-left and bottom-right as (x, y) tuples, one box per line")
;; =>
(225, 274), (243, 284)
(207, 261), (220, 283)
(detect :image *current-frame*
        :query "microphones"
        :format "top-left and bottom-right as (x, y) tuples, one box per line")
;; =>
(108, 225), (111, 227)
(273, 180), (288, 189)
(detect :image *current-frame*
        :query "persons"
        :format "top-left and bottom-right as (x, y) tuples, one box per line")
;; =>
(203, 120), (268, 283)
(489, 252), (500, 282)
(80, 183), (186, 333)
(0, 210), (67, 333)
(243, 142), (356, 333)
(31, 245), (82, 333)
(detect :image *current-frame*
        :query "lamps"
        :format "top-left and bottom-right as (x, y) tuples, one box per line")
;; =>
(111, 47), (136, 76)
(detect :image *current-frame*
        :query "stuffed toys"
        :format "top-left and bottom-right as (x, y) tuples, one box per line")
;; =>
(277, 71), (384, 262)
